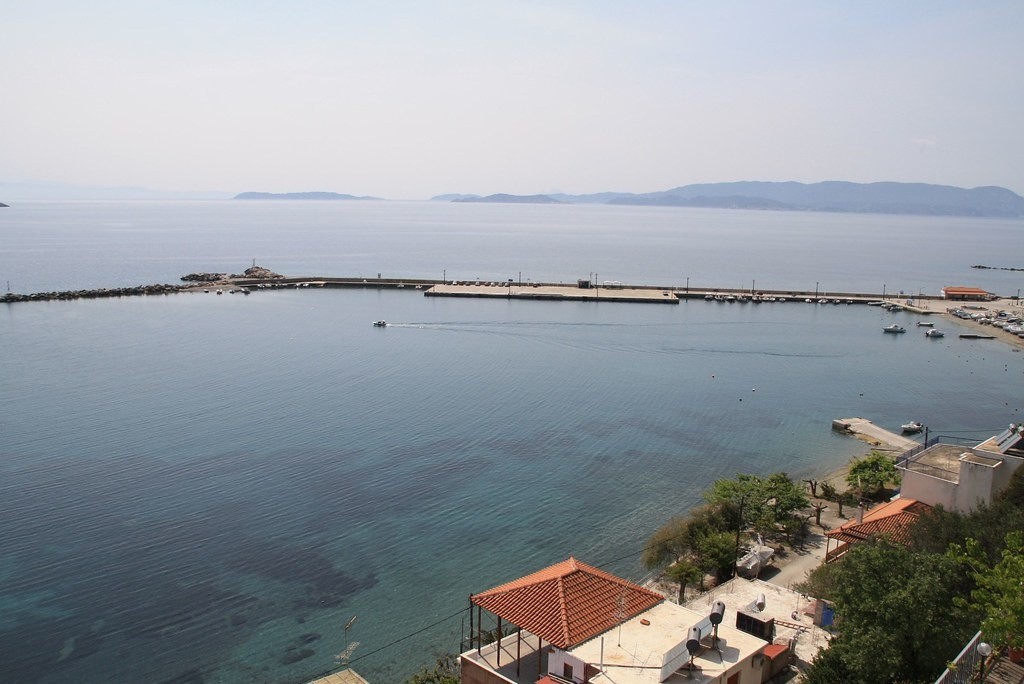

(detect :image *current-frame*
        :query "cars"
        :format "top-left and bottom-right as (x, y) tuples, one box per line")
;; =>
(533, 283), (537, 287)
(447, 281), (509, 287)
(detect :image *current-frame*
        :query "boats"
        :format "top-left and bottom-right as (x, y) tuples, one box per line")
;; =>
(373, 320), (386, 326)
(868, 301), (903, 311)
(818, 299), (828, 303)
(883, 325), (906, 333)
(846, 301), (853, 305)
(705, 295), (785, 303)
(900, 421), (923, 430)
(953, 305), (1024, 338)
(916, 321), (935, 326)
(805, 299), (811, 303)
(926, 329), (944, 336)
(833, 299), (840, 305)
